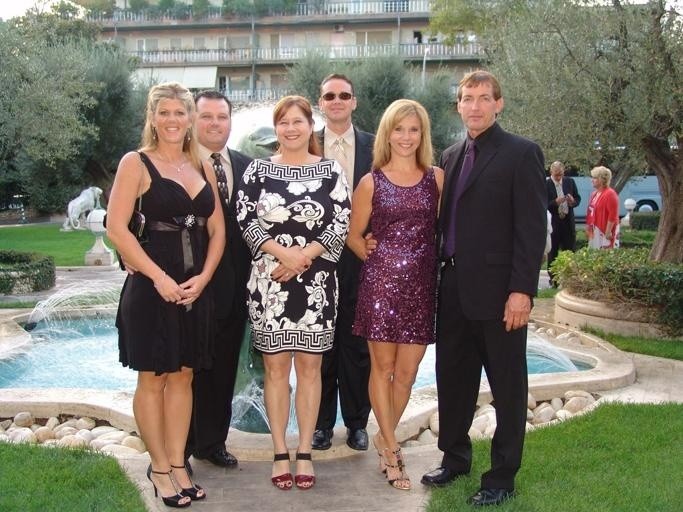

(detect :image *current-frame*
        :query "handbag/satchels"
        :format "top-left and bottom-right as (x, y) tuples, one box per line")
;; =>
(128, 210), (146, 238)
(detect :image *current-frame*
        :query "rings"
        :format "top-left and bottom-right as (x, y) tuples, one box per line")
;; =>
(302, 263), (308, 268)
(284, 273), (288, 276)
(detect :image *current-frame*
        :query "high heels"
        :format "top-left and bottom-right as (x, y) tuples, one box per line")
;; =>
(146, 463), (205, 508)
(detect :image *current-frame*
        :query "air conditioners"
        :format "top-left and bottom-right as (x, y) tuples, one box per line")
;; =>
(333, 23), (344, 32)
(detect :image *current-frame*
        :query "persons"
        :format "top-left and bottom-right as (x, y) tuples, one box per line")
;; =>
(344, 98), (444, 491)
(586, 165), (620, 251)
(183, 88), (254, 477)
(235, 94), (352, 490)
(308, 72), (381, 451)
(362, 69), (548, 507)
(105, 81), (227, 508)
(546, 160), (581, 287)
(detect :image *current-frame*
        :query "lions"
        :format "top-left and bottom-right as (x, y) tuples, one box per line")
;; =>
(67, 187), (103, 230)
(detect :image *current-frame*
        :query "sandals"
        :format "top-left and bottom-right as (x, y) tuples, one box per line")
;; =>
(271, 453), (292, 490)
(373, 437), (411, 490)
(294, 450), (315, 490)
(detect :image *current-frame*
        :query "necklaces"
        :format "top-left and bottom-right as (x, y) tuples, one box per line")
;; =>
(384, 162), (425, 185)
(154, 147), (189, 173)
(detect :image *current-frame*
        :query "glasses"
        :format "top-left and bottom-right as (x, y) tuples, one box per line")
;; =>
(322, 92), (350, 100)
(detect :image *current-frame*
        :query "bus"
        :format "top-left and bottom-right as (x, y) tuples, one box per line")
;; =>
(7, 192), (26, 210)
(543, 141), (678, 222)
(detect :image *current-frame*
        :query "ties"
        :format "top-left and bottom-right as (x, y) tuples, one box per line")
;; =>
(334, 137), (346, 175)
(447, 139), (475, 255)
(210, 153), (229, 207)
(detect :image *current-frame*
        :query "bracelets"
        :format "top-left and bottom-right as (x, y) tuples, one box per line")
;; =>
(305, 242), (316, 260)
(152, 270), (166, 289)
(604, 232), (611, 238)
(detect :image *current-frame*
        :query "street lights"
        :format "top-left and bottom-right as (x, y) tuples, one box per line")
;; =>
(420, 48), (431, 86)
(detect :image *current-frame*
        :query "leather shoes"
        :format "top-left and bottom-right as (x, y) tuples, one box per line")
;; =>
(205, 450), (236, 465)
(346, 428), (368, 450)
(465, 488), (516, 507)
(311, 429), (332, 450)
(420, 467), (470, 487)
